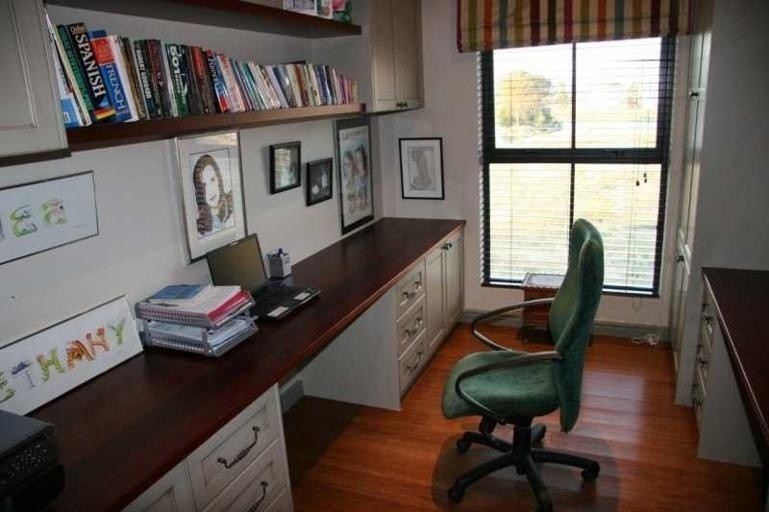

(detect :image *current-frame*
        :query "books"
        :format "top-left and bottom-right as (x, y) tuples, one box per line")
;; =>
(296, 61), (357, 106)
(205, 50), (302, 112)
(132, 282), (256, 352)
(52, 21), (217, 128)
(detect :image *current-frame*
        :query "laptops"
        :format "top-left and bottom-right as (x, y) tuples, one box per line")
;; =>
(205, 232), (321, 319)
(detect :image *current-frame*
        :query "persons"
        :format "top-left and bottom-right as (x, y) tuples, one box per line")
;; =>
(344, 144), (369, 214)
(194, 155), (233, 236)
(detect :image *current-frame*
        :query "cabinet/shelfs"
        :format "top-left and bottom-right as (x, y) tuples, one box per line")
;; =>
(43, 1), (366, 154)
(395, 227), (463, 411)
(694, 268), (768, 468)
(0, 0), (67, 157)
(369, 0), (426, 112)
(667, 0), (768, 409)
(126, 384), (294, 512)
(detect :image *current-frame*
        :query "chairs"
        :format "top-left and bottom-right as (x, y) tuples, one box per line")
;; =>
(442, 217), (604, 512)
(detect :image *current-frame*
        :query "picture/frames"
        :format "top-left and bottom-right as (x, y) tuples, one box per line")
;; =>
(0, 169), (100, 264)
(398, 136), (445, 200)
(305, 157), (333, 206)
(331, 117), (375, 236)
(268, 141), (301, 195)
(0, 295), (148, 416)
(173, 128), (248, 265)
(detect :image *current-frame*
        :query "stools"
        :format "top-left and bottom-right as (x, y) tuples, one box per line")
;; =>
(520, 272), (566, 338)
